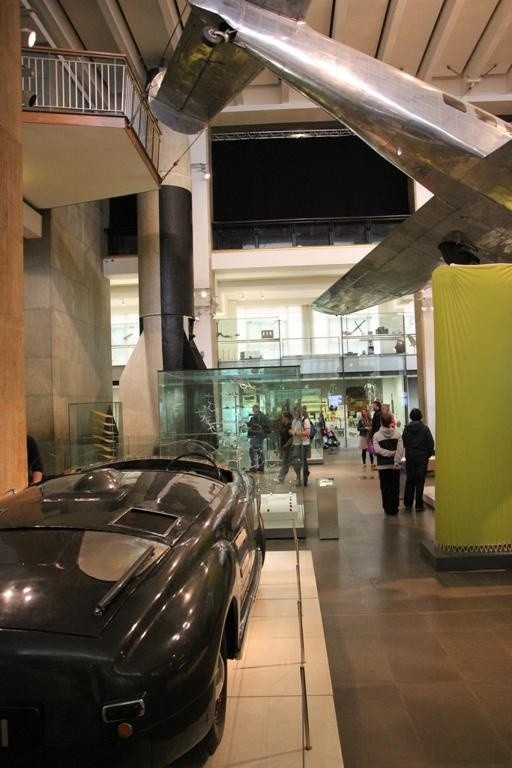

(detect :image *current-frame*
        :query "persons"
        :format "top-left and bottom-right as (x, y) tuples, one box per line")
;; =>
(381, 403), (396, 429)
(357, 407), (376, 468)
(274, 403), (315, 486)
(401, 408), (435, 511)
(26, 435), (44, 486)
(373, 414), (404, 515)
(245, 404), (267, 472)
(368, 401), (381, 440)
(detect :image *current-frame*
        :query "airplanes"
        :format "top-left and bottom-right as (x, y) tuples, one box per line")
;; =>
(144, 0), (511, 323)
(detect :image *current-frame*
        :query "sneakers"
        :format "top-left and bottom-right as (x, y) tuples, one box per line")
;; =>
(249, 466), (264, 472)
(406, 507), (425, 513)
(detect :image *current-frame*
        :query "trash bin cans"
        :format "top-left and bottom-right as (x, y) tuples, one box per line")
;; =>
(316, 477), (339, 540)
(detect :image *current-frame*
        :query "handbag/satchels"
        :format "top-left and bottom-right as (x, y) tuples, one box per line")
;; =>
(309, 421), (315, 439)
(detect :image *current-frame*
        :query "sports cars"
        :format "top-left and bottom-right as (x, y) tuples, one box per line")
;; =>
(0, 438), (269, 768)
